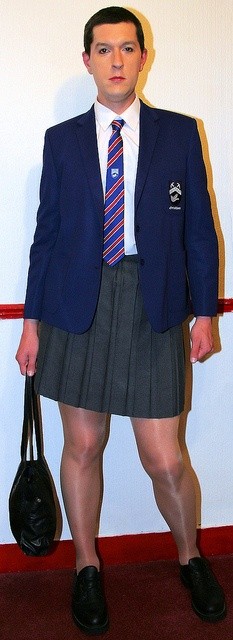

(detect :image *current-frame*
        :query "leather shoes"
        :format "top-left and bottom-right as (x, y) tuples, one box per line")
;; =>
(70, 565), (109, 635)
(178, 553), (226, 623)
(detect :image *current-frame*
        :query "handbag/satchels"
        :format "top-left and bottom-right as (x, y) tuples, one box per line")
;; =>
(8, 363), (62, 557)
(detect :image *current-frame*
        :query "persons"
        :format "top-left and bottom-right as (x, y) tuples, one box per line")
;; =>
(14, 6), (226, 633)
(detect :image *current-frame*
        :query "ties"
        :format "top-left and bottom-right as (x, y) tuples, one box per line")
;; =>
(101, 118), (126, 268)
